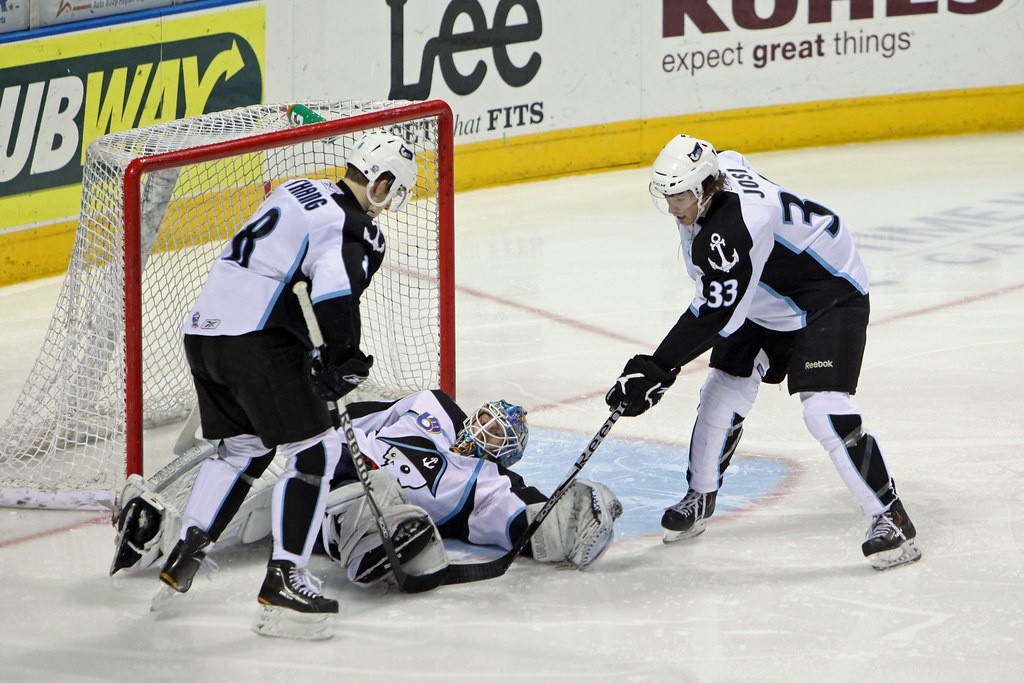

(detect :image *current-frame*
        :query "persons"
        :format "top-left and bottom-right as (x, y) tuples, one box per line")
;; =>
(147, 132), (417, 641)
(108, 390), (622, 587)
(606, 134), (921, 569)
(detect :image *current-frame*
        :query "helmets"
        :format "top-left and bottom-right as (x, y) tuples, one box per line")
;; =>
(648, 133), (720, 198)
(346, 130), (419, 212)
(450, 398), (530, 469)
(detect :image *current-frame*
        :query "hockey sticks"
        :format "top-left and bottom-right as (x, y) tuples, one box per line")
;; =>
(292, 281), (453, 594)
(173, 398), (203, 457)
(444, 394), (630, 585)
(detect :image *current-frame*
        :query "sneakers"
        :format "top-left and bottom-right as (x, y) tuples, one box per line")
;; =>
(108, 473), (168, 576)
(149, 524), (212, 616)
(661, 487), (719, 544)
(860, 499), (923, 572)
(254, 559), (340, 640)
(353, 518), (435, 588)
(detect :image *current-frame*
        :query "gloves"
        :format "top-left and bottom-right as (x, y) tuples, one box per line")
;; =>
(605, 354), (681, 418)
(308, 337), (374, 403)
(525, 478), (624, 568)
